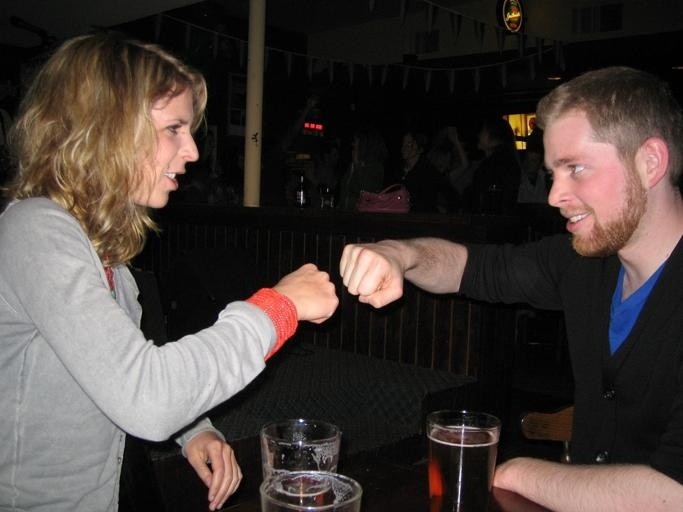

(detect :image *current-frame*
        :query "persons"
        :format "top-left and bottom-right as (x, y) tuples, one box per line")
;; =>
(206, 117), (550, 213)
(1, 30), (339, 511)
(338, 63), (683, 512)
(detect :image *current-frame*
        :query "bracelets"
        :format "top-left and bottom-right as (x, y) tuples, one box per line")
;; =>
(243, 286), (298, 363)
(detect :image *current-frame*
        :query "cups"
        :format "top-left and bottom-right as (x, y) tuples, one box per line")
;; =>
(259, 418), (363, 510)
(426, 410), (502, 512)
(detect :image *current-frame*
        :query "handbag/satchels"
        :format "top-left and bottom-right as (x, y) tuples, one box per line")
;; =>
(355, 183), (411, 215)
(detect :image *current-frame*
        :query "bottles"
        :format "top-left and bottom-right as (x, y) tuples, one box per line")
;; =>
(295, 168), (309, 209)
